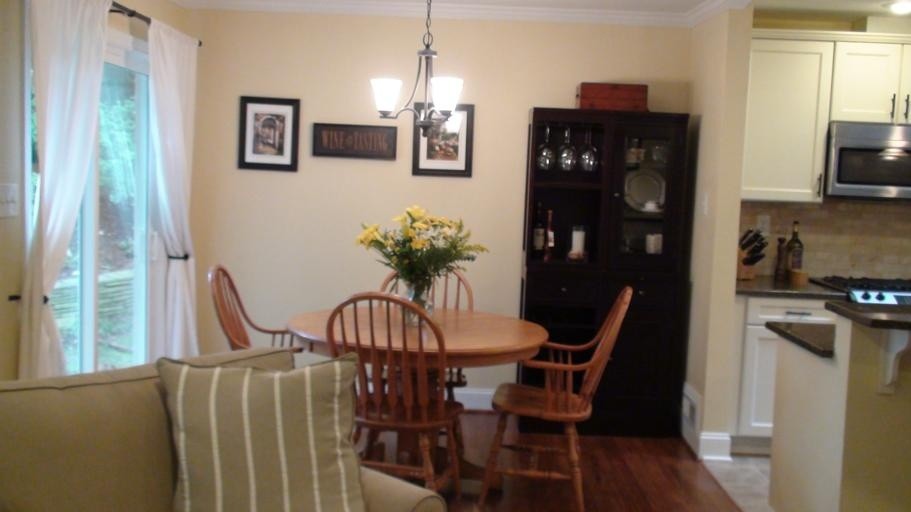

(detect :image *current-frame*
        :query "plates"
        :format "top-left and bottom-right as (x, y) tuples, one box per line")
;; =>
(625, 168), (666, 212)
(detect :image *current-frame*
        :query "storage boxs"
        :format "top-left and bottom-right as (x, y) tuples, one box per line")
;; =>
(575, 80), (649, 111)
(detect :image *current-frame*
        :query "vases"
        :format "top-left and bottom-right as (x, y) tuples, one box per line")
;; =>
(400, 274), (435, 326)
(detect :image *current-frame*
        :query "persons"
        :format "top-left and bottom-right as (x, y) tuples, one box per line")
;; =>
(254, 113), (286, 158)
(428, 110), (453, 159)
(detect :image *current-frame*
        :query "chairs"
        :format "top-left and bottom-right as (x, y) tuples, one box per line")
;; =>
(207, 261), (304, 371)
(376, 265), (475, 472)
(324, 292), (467, 504)
(476, 283), (637, 510)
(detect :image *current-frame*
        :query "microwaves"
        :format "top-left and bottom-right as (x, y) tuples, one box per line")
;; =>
(826, 122), (911, 202)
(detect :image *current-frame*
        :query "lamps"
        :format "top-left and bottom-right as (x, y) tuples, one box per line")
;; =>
(366, 3), (469, 140)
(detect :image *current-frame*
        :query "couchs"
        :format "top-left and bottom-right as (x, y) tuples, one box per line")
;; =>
(1, 342), (450, 510)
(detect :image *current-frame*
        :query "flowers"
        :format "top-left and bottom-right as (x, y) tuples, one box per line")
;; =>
(355, 204), (489, 304)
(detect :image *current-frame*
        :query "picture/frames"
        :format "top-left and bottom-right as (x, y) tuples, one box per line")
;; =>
(235, 94), (302, 173)
(309, 121), (399, 161)
(412, 100), (474, 179)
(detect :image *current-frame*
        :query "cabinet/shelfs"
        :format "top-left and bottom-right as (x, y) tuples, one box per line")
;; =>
(739, 32), (838, 205)
(831, 29), (910, 126)
(738, 295), (841, 457)
(515, 102), (692, 441)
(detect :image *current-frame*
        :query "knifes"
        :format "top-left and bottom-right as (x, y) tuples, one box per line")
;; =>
(741, 229), (769, 266)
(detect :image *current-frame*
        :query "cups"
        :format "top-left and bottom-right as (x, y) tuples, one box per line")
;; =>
(789, 270), (809, 289)
(645, 233), (665, 255)
(571, 231), (586, 256)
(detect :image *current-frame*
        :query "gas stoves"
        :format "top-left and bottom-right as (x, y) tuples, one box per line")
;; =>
(809, 274), (911, 304)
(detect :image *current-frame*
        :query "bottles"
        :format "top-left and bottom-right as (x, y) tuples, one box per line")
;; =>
(626, 137), (664, 167)
(787, 220), (805, 269)
(775, 238), (788, 282)
(535, 124), (598, 171)
(535, 203), (557, 251)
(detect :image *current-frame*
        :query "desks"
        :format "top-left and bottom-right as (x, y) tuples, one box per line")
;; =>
(285, 306), (552, 487)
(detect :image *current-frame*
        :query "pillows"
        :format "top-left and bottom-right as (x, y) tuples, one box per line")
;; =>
(157, 352), (367, 510)
